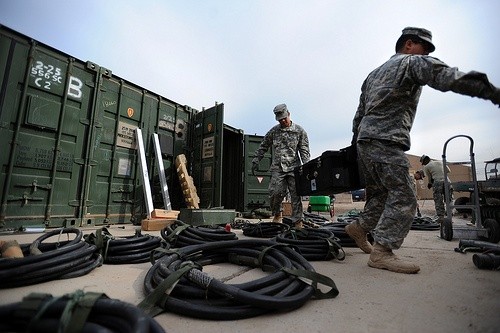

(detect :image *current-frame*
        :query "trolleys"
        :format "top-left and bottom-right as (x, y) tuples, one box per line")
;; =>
(439, 134), (500, 244)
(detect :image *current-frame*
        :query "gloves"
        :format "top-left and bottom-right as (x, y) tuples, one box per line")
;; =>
(252, 162), (259, 175)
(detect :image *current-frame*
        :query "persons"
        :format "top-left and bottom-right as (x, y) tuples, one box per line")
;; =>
(251, 104), (310, 228)
(350, 27), (500, 273)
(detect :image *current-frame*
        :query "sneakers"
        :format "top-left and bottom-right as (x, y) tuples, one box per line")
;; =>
(272, 212), (282, 223)
(295, 219), (303, 228)
(368, 244), (421, 274)
(437, 215), (444, 223)
(344, 220), (372, 253)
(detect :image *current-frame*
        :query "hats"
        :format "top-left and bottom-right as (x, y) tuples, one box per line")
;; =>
(402, 26), (435, 52)
(420, 154), (426, 165)
(418, 169), (425, 180)
(273, 104), (288, 121)
